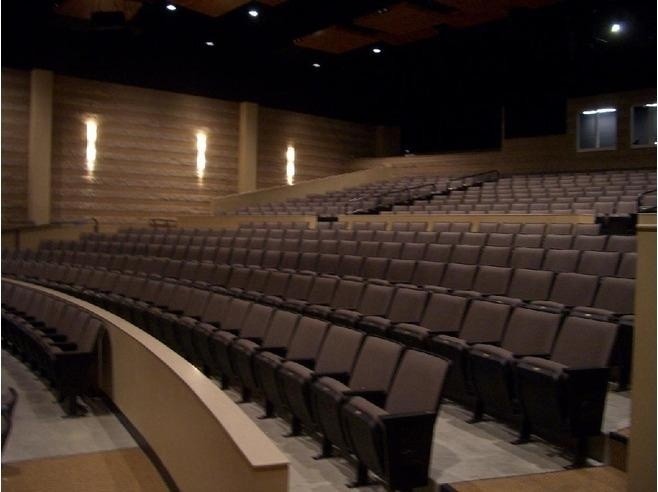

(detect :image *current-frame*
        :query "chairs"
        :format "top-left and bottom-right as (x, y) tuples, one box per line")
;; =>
(225, 172), (655, 217)
(1, 221), (639, 492)
